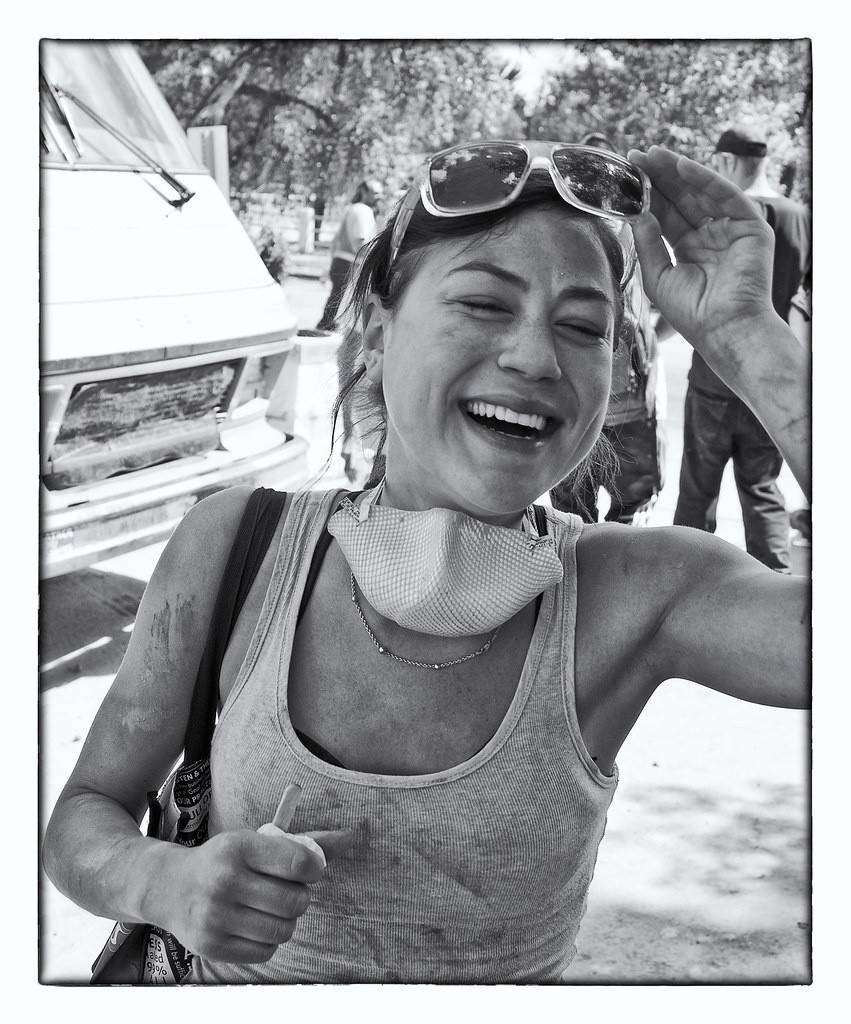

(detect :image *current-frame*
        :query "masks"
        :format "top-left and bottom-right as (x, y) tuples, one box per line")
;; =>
(328, 479), (566, 640)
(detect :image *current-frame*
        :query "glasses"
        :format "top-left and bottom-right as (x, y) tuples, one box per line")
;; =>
(384, 140), (651, 293)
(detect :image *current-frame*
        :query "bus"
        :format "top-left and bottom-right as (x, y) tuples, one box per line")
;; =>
(44, 41), (307, 576)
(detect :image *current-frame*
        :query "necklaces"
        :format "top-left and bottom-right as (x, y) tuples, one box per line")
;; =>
(351, 573), (503, 668)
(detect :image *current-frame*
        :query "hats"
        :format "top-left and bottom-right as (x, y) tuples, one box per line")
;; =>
(711, 129), (767, 158)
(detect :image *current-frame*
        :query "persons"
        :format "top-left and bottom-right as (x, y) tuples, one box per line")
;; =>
(550, 221), (677, 524)
(318, 179), (382, 331)
(39, 145), (811, 982)
(654, 126), (811, 575)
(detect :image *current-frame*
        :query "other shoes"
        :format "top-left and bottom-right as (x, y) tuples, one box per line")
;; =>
(315, 322), (339, 332)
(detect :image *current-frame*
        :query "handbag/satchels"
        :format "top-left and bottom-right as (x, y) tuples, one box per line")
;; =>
(89, 487), (287, 986)
